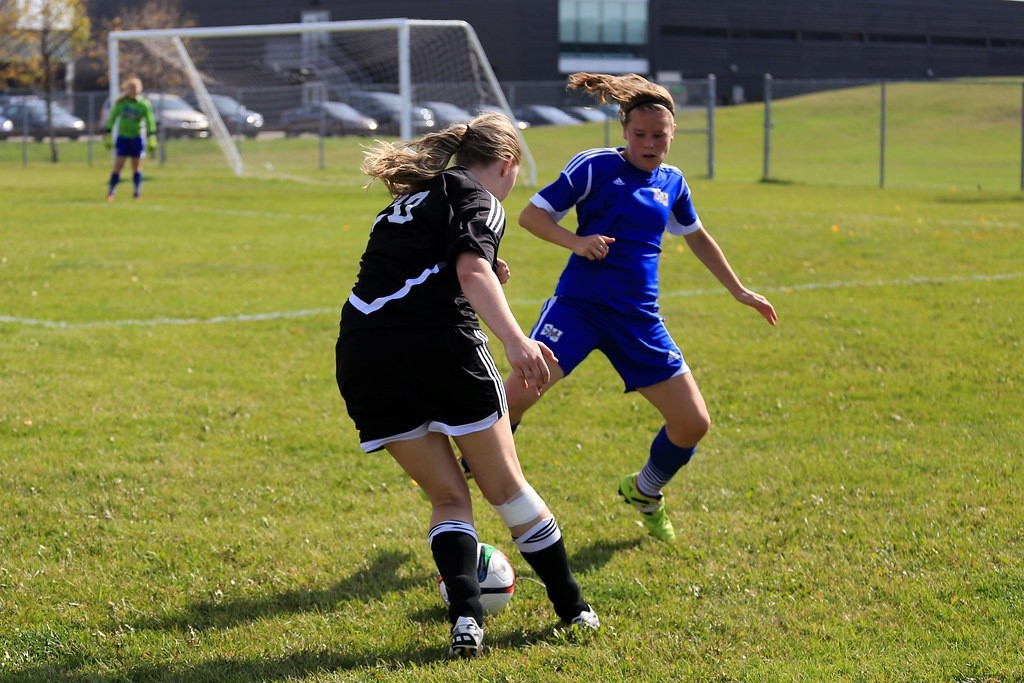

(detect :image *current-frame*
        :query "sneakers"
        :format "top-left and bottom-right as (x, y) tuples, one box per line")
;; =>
(417, 452), (474, 500)
(447, 615), (484, 655)
(571, 601), (601, 636)
(618, 472), (674, 543)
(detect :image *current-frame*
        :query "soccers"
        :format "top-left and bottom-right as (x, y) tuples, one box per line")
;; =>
(436, 540), (518, 617)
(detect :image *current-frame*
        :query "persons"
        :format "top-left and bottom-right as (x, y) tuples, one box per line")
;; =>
(418, 72), (778, 543)
(335, 112), (601, 659)
(103, 79), (157, 202)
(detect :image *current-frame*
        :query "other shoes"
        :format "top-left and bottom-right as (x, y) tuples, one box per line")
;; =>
(105, 192), (114, 200)
(132, 193), (141, 201)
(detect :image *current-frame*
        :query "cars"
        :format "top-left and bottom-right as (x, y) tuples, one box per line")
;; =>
(187, 94), (265, 139)
(145, 94), (211, 137)
(346, 91), (434, 137)
(275, 101), (373, 136)
(0, 93), (84, 142)
(422, 100), (627, 134)
